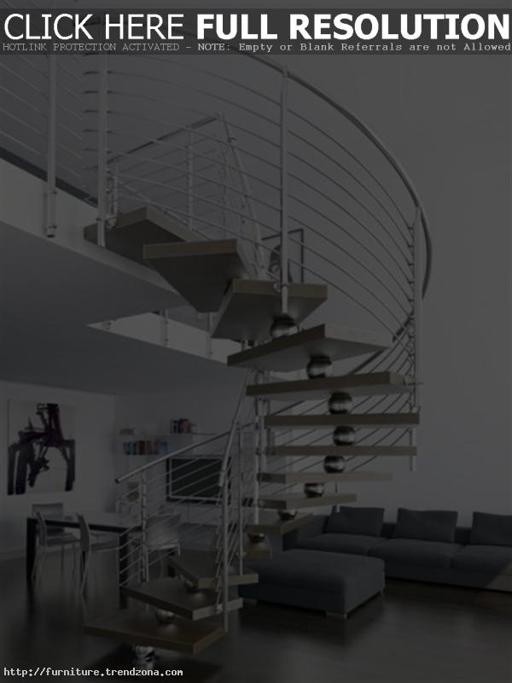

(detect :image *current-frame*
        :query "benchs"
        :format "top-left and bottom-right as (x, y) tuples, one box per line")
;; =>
(239, 546), (386, 619)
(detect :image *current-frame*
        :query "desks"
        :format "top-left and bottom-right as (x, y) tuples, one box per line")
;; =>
(26, 511), (176, 610)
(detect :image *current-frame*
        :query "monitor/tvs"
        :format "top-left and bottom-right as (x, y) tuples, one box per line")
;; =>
(165, 454), (231, 503)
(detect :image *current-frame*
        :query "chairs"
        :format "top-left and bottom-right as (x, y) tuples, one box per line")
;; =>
(31, 501), (180, 600)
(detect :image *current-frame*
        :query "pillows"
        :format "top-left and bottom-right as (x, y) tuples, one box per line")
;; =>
(323, 504), (512, 546)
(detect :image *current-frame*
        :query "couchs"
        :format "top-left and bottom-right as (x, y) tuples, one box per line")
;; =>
(282, 512), (511, 594)
